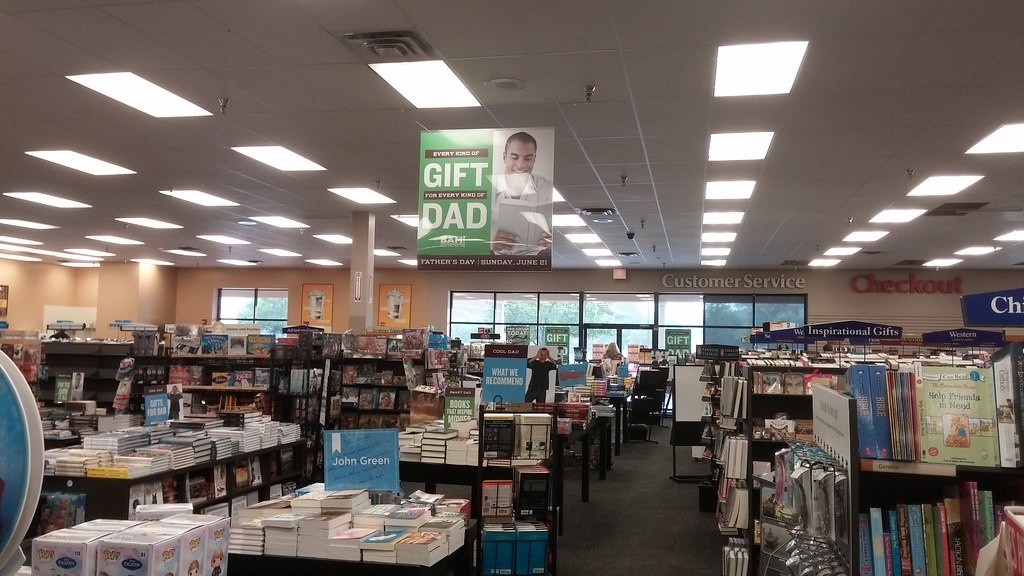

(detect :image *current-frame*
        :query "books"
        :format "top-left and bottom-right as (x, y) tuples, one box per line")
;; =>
(840, 336), (1023, 467)
(481, 403), (552, 576)
(325, 328), (428, 359)
(848, 478), (1024, 575)
(559, 362), (634, 398)
(32, 396), (302, 529)
(337, 386), (410, 410)
(715, 428), (749, 479)
(718, 374), (748, 418)
(73, 388), (83, 399)
(465, 342), (485, 375)
(339, 361), (408, 386)
(222, 480), (471, 569)
(742, 345), (837, 368)
(393, 414), (478, 467)
(720, 537), (748, 576)
(554, 397), (617, 437)
(496, 195), (552, 247)
(336, 410), (394, 430)
(716, 478), (750, 531)
(425, 347), (448, 389)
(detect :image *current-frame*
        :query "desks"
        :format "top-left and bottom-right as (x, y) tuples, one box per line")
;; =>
(227, 518), (479, 576)
(399, 461), (482, 526)
(556, 391), (630, 536)
(21, 438), (304, 535)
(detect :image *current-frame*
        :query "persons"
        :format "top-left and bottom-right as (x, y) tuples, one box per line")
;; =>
(72, 374), (83, 400)
(166, 386), (182, 421)
(526, 347), (557, 403)
(491, 129), (551, 258)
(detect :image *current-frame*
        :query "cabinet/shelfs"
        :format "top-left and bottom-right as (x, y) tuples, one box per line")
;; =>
(41, 328), (483, 488)
(696, 345), (1023, 576)
(473, 405), (557, 575)
